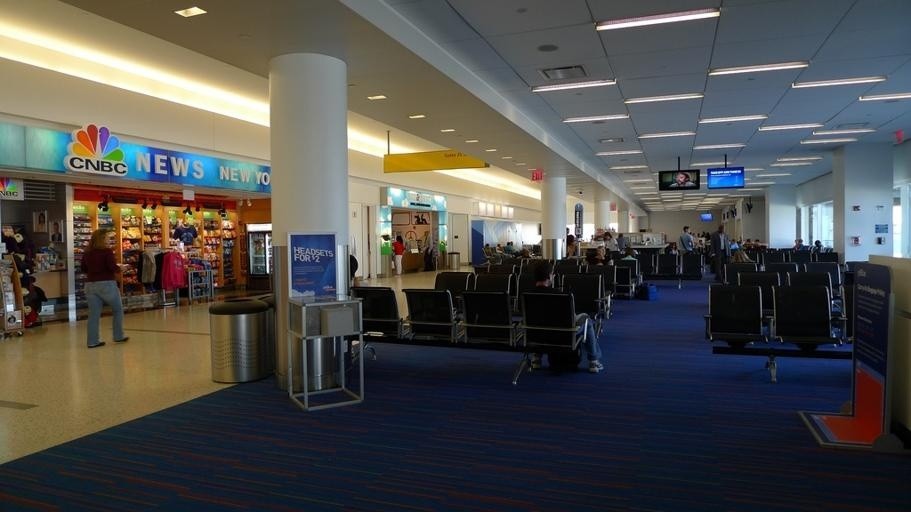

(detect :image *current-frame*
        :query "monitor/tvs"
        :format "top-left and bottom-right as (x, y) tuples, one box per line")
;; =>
(659, 169), (701, 191)
(708, 167), (745, 189)
(700, 213), (714, 222)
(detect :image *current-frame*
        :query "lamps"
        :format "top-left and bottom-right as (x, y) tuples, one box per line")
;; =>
(97, 194), (113, 212)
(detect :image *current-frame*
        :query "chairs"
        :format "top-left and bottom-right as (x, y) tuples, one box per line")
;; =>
(703, 242), (871, 386)
(434, 241), (705, 339)
(350, 284), (591, 386)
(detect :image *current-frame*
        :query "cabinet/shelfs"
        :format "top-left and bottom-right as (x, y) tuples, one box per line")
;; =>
(74, 199), (241, 320)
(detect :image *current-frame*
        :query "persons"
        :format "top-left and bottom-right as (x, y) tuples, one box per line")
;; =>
(391, 236), (406, 274)
(36, 213), (45, 231)
(679, 226), (694, 255)
(520, 247), (531, 258)
(538, 239), (542, 248)
(670, 172), (696, 186)
(171, 219), (202, 248)
(812, 240), (824, 257)
(527, 260), (605, 374)
(567, 228), (581, 273)
(420, 230), (434, 272)
(80, 228), (130, 348)
(731, 235), (760, 262)
(669, 242), (679, 255)
(793, 238), (803, 251)
(586, 228), (635, 266)
(689, 231), (710, 255)
(51, 221), (62, 241)
(713, 225), (732, 283)
(484, 241), (516, 264)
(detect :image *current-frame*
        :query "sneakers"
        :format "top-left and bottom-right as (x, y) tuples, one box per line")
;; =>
(530, 358), (542, 370)
(589, 361), (605, 373)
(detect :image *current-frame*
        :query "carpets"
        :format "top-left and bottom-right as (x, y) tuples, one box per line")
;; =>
(0, 276), (907, 510)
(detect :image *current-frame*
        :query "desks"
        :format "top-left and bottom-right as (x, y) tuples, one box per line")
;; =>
(287, 293), (366, 412)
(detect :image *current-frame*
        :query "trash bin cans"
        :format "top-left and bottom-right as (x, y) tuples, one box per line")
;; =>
(208, 300), (274, 383)
(448, 252), (460, 270)
